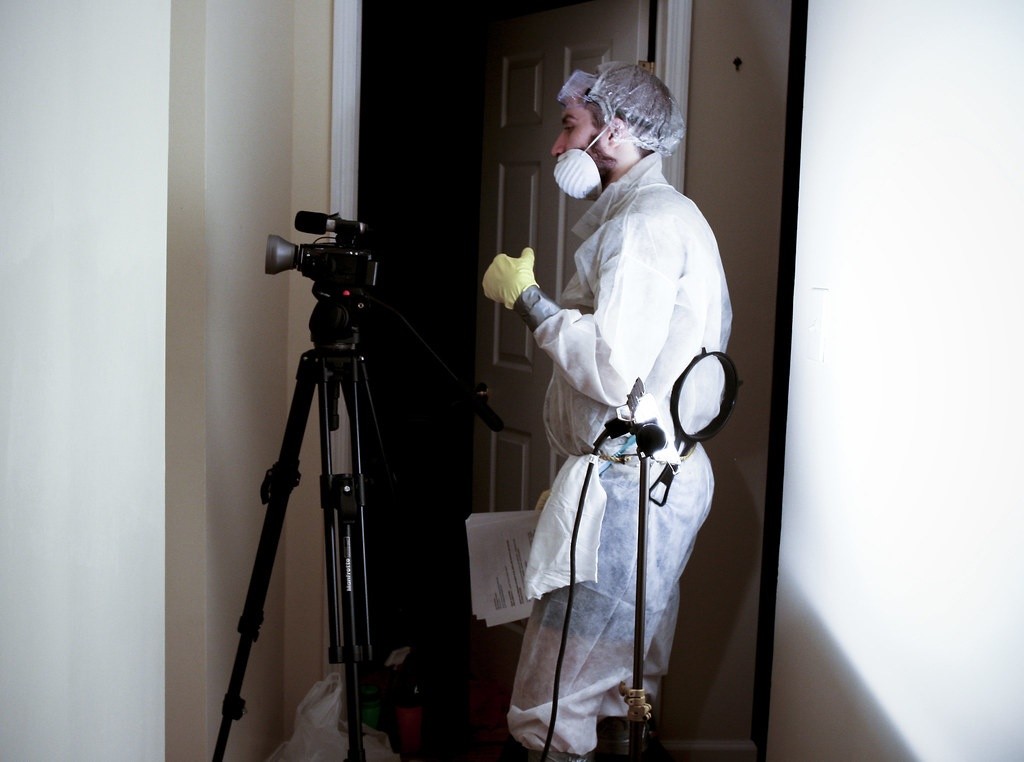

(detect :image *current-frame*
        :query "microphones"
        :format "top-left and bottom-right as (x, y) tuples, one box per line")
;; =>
(294, 210), (366, 235)
(637, 424), (667, 453)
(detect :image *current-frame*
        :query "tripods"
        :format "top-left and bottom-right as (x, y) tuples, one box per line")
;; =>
(212, 286), (505, 762)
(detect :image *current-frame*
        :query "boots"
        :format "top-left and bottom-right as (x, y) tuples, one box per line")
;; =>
(597, 716), (648, 762)
(528, 750), (595, 762)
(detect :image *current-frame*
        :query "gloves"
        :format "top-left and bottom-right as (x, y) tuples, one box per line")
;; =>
(482, 247), (540, 309)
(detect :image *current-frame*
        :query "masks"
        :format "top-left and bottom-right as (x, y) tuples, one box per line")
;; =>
(554, 126), (609, 200)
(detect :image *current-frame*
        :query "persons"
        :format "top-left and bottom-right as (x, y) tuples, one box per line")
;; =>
(479, 58), (729, 762)
